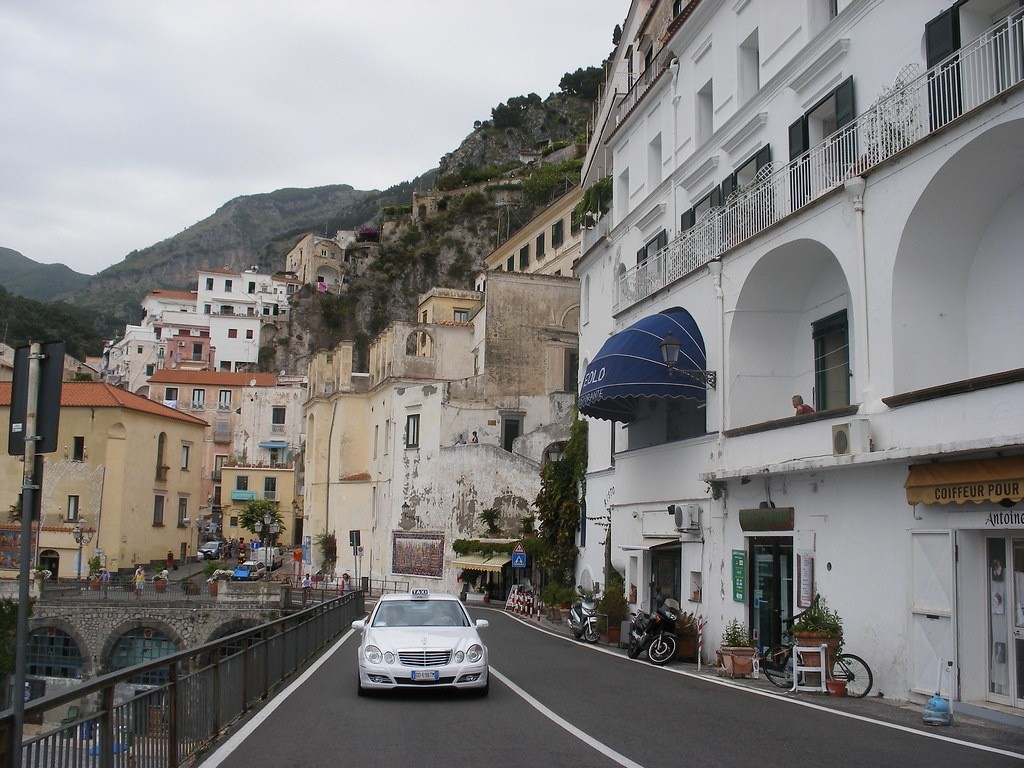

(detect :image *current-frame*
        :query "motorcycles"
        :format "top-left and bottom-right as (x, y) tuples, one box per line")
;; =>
(568, 590), (601, 643)
(628, 598), (682, 665)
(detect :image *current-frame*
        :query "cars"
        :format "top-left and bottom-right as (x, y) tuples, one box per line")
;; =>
(196, 551), (205, 560)
(350, 585), (490, 699)
(199, 540), (229, 559)
(230, 559), (268, 581)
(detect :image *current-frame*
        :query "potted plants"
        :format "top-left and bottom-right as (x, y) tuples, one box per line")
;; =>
(826, 645), (847, 697)
(596, 582), (629, 642)
(477, 508), (504, 539)
(88, 555), (102, 591)
(539, 584), (577, 623)
(518, 515), (538, 540)
(789, 601), (851, 696)
(122, 576), (136, 592)
(182, 578), (201, 595)
(718, 619), (754, 678)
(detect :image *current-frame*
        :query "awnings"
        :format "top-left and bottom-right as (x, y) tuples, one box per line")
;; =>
(616, 537), (681, 552)
(450, 553), (512, 573)
(259, 441), (289, 462)
(577, 307), (707, 425)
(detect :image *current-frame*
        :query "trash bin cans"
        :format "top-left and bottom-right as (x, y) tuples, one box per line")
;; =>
(620, 621), (636, 649)
(362, 577), (368, 591)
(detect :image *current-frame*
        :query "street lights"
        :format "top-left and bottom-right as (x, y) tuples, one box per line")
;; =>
(72, 517), (95, 592)
(252, 511), (281, 579)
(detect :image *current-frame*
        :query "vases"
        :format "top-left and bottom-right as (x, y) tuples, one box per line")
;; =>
(207, 582), (218, 596)
(217, 574), (227, 580)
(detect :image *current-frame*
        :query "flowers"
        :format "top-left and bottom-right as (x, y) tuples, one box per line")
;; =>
(213, 568), (233, 577)
(205, 577), (214, 585)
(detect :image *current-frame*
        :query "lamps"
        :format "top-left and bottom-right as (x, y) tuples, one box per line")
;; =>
(741, 476), (752, 485)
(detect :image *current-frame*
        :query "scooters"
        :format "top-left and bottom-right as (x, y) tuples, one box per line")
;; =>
(236, 547), (246, 563)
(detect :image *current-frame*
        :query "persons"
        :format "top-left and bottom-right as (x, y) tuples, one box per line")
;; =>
(339, 572), (352, 596)
(96, 569), (110, 582)
(454, 433), (467, 445)
(992, 592), (1003, 608)
(299, 573), (312, 596)
(162, 567), (169, 585)
(992, 559), (1003, 577)
(223, 536), (246, 552)
(995, 645), (1005, 663)
(129, 564), (145, 595)
(792, 395), (815, 416)
(167, 551), (174, 568)
(470, 431), (478, 443)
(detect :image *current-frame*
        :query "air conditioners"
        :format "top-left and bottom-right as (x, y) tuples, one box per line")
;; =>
(674, 504), (698, 529)
(178, 341), (185, 346)
(831, 419), (871, 457)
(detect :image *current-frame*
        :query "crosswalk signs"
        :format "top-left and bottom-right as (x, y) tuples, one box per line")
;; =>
(512, 553), (528, 567)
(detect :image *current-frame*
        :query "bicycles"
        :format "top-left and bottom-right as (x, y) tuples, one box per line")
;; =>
(761, 593), (873, 698)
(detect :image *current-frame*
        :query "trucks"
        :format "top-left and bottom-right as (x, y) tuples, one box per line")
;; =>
(248, 545), (284, 571)
